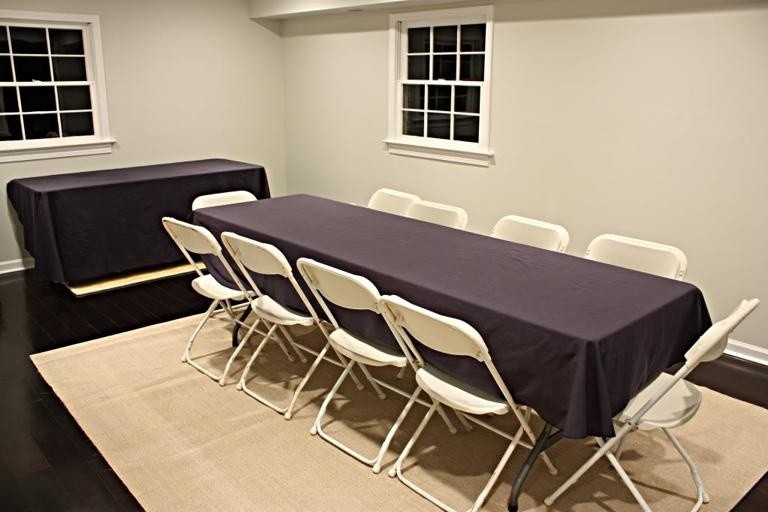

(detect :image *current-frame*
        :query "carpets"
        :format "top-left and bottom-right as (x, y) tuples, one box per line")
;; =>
(27, 305), (767, 512)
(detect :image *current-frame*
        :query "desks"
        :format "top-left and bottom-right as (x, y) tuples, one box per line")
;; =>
(183, 193), (715, 512)
(13, 158), (262, 288)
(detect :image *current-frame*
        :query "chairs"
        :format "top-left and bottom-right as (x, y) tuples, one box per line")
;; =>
(584, 233), (688, 283)
(190, 190), (261, 338)
(161, 216), (295, 389)
(296, 256), (460, 476)
(492, 214), (571, 254)
(376, 292), (559, 512)
(543, 295), (762, 512)
(405, 200), (470, 233)
(367, 187), (420, 217)
(220, 230), (365, 421)
